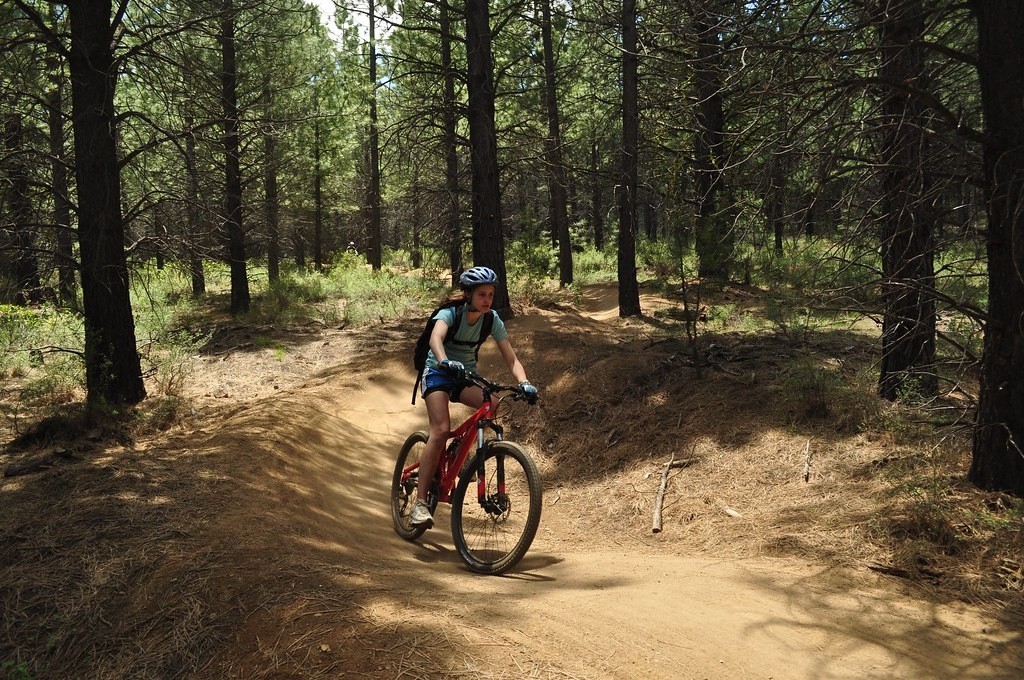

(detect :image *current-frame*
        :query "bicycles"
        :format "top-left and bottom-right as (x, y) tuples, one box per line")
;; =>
(389, 360), (548, 575)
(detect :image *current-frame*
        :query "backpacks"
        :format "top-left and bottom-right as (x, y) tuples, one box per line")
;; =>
(413, 302), (495, 371)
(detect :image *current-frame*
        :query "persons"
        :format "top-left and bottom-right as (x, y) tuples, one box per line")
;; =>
(405, 265), (539, 529)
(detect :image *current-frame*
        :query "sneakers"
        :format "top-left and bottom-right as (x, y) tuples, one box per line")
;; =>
(409, 501), (435, 527)
(453, 451), (476, 482)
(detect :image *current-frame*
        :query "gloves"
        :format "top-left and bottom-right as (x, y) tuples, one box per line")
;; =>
(519, 383), (538, 401)
(442, 360), (463, 372)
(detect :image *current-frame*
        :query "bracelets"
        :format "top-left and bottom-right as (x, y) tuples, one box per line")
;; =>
(519, 380), (530, 386)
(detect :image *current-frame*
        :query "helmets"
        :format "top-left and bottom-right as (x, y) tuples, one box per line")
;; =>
(459, 266), (498, 288)
